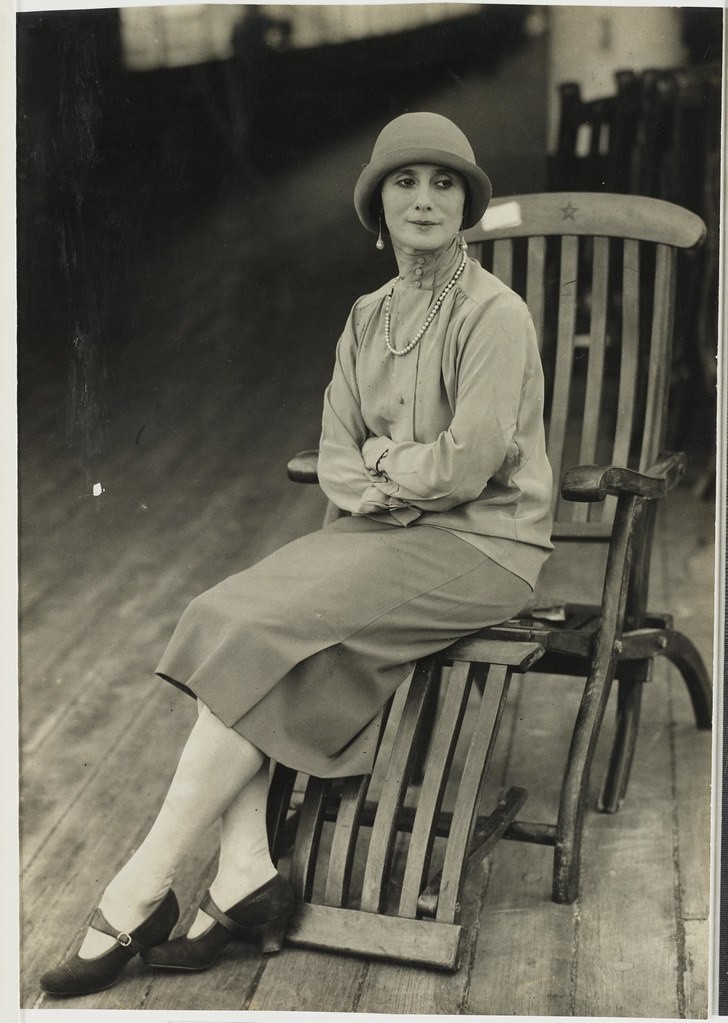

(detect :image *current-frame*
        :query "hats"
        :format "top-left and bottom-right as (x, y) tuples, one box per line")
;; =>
(352, 112), (493, 235)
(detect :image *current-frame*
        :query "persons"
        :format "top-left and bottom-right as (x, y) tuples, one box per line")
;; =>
(39, 112), (553, 995)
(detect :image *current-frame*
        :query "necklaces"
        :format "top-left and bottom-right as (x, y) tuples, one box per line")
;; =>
(385, 251), (467, 356)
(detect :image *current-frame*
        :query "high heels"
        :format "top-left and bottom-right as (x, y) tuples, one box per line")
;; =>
(141, 872), (296, 970)
(39, 887), (180, 998)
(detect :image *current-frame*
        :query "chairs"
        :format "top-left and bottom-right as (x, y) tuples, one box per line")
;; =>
(263, 192), (711, 967)
(530, 61), (719, 432)
